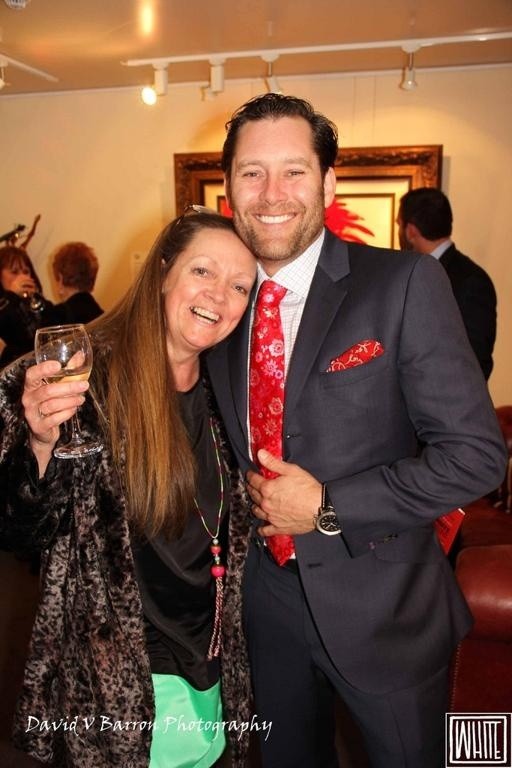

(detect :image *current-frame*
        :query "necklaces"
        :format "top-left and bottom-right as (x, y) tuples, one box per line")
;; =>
(176, 420), (225, 662)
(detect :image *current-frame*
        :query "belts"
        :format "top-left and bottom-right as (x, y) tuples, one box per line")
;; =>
(264, 547), (298, 570)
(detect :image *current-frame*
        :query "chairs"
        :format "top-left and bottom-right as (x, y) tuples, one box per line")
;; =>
(449, 404), (512, 708)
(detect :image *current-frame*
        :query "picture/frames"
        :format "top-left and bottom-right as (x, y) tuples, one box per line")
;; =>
(169, 143), (441, 261)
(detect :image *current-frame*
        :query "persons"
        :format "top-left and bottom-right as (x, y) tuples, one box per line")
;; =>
(0, 201), (261, 768)
(0, 245), (41, 367)
(0, 240), (105, 370)
(394, 185), (498, 386)
(204, 92), (509, 768)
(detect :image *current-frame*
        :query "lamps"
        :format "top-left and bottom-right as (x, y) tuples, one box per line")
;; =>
(140, 46), (420, 106)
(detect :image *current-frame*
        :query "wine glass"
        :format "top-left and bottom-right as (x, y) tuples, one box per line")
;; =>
(33, 322), (104, 462)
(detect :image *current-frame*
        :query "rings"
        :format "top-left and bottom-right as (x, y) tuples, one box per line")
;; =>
(38, 403), (48, 417)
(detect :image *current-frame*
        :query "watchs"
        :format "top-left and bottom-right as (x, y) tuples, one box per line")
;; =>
(313, 481), (342, 538)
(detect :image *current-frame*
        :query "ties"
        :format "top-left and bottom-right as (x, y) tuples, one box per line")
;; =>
(248, 283), (294, 568)
